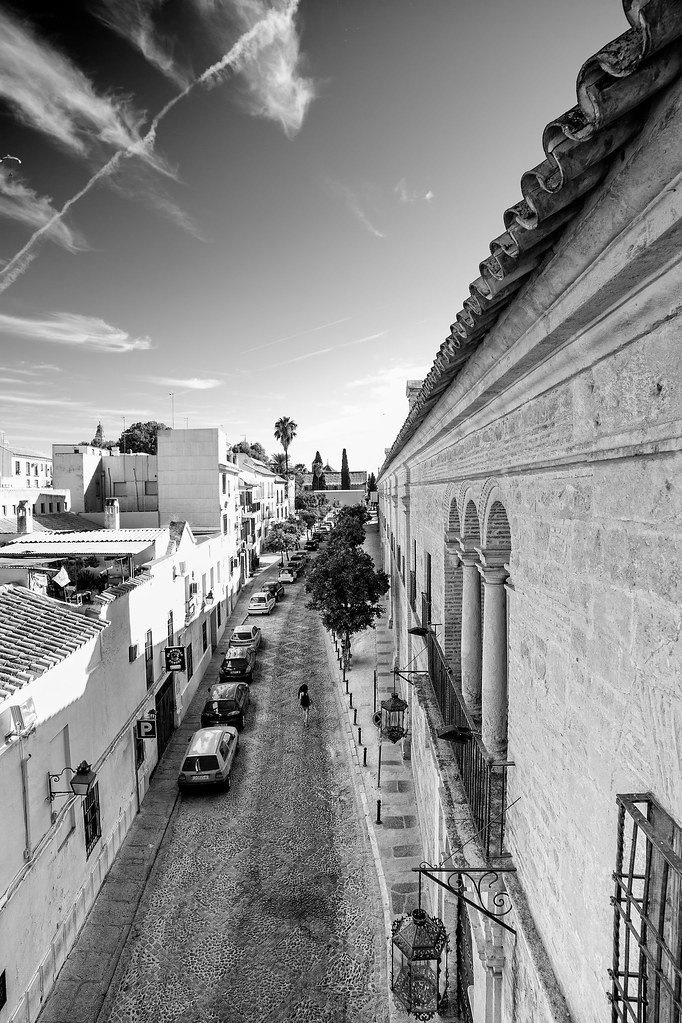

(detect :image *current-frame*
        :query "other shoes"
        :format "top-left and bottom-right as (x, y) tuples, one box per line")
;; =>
(303, 721), (309, 728)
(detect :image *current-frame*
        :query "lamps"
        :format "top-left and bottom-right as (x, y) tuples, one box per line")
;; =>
(387, 908), (451, 1023)
(43, 760), (98, 803)
(201, 590), (215, 610)
(380, 692), (410, 744)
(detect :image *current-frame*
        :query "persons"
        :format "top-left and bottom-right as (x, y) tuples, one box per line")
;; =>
(298, 684), (310, 728)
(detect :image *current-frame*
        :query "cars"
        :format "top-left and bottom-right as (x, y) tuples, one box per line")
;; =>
(288, 507), (342, 577)
(278, 567), (298, 583)
(201, 680), (250, 730)
(219, 646), (257, 682)
(262, 581), (284, 602)
(248, 593), (277, 615)
(229, 624), (263, 647)
(177, 724), (240, 796)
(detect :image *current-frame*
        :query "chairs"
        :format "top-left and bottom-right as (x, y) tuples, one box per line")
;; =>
(64, 585), (84, 607)
